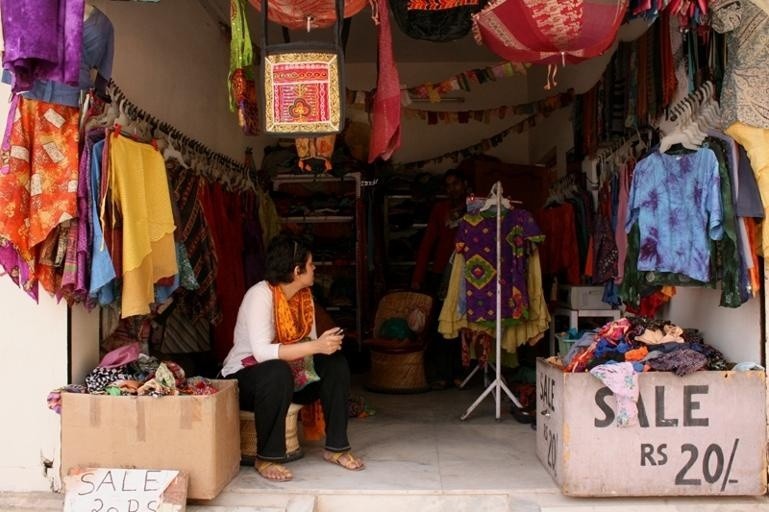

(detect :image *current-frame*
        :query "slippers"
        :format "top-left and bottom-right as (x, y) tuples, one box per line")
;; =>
(254, 458), (294, 482)
(321, 448), (366, 471)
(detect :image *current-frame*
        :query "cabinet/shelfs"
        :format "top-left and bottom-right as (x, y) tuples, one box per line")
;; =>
(547, 306), (622, 359)
(383, 192), (451, 267)
(271, 168), (367, 342)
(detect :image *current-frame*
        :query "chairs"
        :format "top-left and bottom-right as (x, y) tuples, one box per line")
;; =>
(360, 291), (434, 395)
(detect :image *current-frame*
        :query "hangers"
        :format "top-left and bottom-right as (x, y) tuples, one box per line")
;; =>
(84, 82), (264, 195)
(480, 181), (513, 212)
(544, 173), (579, 207)
(659, 79), (725, 153)
(597, 124), (648, 187)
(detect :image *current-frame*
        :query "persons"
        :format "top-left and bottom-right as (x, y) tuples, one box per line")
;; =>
(216, 230), (364, 481)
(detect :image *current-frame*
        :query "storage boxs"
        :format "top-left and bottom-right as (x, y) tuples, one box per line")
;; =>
(553, 332), (581, 359)
(533, 356), (769, 499)
(58, 376), (244, 503)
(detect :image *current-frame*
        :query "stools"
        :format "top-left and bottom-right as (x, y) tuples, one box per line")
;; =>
(236, 400), (306, 469)
